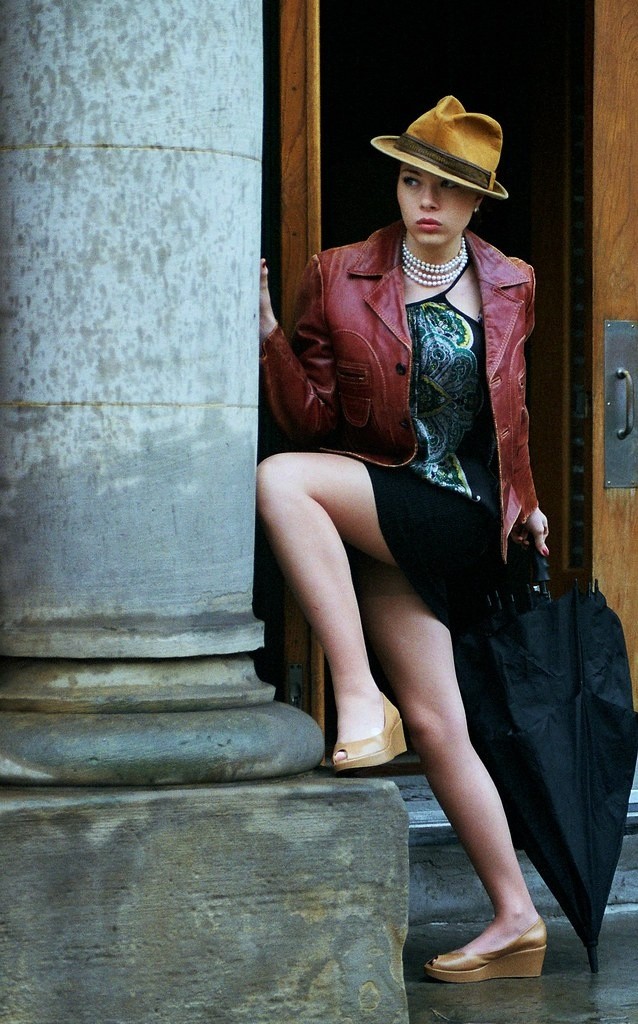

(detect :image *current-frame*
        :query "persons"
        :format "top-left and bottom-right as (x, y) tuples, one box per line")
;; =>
(257, 95), (550, 983)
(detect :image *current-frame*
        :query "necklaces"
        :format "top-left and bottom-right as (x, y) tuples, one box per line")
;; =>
(402, 236), (468, 286)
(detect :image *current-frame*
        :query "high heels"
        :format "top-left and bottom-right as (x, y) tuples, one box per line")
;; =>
(425, 915), (547, 982)
(333, 693), (407, 772)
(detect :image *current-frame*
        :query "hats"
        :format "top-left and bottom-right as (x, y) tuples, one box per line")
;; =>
(371, 95), (508, 199)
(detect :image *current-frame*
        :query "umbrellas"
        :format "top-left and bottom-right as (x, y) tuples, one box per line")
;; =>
(453, 553), (638, 973)
